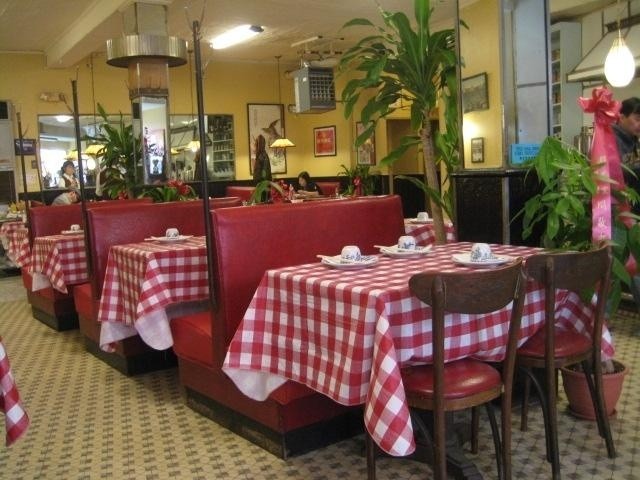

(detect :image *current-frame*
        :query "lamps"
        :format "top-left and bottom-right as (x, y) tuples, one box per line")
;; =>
(85, 54), (107, 154)
(269, 55), (295, 148)
(185, 50), (200, 152)
(604, 0), (637, 88)
(64, 149), (78, 161)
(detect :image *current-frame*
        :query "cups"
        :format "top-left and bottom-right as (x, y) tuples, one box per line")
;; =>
(340, 245), (361, 263)
(470, 243), (492, 262)
(417, 212), (428, 221)
(397, 236), (415, 251)
(166, 228), (179, 237)
(168, 170), (193, 181)
(71, 224), (80, 232)
(242, 201), (254, 206)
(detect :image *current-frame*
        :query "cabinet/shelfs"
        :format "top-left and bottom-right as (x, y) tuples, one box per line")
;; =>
(213, 139), (233, 178)
(551, 21), (582, 148)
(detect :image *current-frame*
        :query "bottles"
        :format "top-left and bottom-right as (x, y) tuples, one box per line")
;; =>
(11, 199), (31, 215)
(253, 135), (272, 203)
(207, 117), (234, 172)
(446, 223), (454, 244)
(288, 184), (294, 200)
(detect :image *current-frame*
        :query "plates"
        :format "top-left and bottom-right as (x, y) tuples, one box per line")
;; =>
(60, 230), (84, 235)
(452, 254), (513, 269)
(408, 219), (433, 224)
(291, 199), (304, 203)
(152, 236), (193, 244)
(295, 194), (306, 199)
(321, 256), (376, 271)
(381, 249), (428, 259)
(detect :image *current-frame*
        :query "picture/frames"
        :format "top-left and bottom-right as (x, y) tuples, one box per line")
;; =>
(14, 139), (36, 155)
(313, 125), (337, 157)
(460, 72), (489, 114)
(470, 138), (484, 163)
(247, 103), (287, 176)
(356, 120), (377, 166)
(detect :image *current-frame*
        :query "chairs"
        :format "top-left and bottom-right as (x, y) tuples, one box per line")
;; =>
(366, 256), (528, 480)
(516, 244), (616, 480)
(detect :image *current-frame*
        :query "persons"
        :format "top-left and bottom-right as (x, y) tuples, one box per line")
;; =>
(294, 171), (322, 195)
(58, 160), (80, 190)
(50, 185), (82, 205)
(193, 151), (208, 180)
(611, 97), (640, 312)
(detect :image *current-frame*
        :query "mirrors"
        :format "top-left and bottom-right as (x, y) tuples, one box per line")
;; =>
(142, 96), (170, 186)
(131, 96), (145, 186)
(37, 114), (235, 191)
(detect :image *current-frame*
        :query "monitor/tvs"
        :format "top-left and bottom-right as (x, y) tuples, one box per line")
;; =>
(0, 100), (15, 119)
(132, 103), (140, 120)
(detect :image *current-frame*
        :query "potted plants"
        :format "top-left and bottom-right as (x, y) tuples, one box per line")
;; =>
(510, 137), (640, 417)
